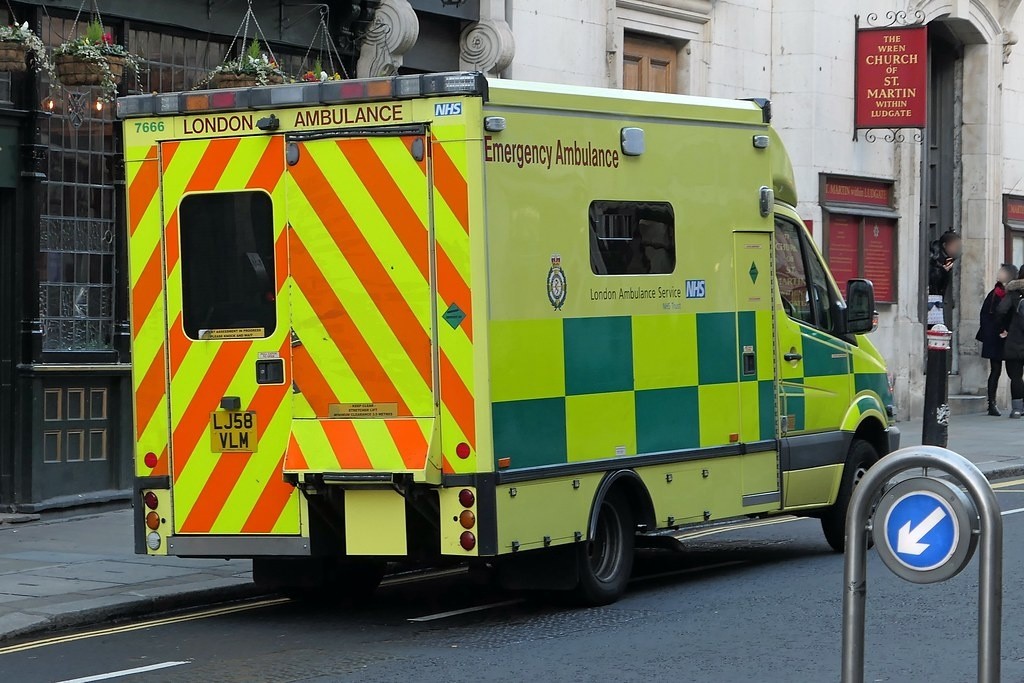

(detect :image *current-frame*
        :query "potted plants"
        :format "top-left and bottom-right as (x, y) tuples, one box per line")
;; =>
(54, 51), (127, 85)
(215, 70), (286, 90)
(0, 40), (29, 73)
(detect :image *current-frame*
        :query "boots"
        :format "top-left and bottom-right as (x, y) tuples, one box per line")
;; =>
(1009, 398), (1023, 418)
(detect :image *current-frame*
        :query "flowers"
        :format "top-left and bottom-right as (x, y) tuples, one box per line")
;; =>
(297, 64), (344, 82)
(54, 20), (129, 61)
(214, 52), (285, 79)
(0, 21), (56, 85)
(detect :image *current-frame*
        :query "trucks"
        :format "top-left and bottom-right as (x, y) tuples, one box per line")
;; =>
(111, 69), (903, 616)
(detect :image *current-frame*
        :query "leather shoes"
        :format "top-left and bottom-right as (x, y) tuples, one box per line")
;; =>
(986, 403), (1002, 416)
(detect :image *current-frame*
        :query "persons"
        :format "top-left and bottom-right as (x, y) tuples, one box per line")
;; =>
(928, 229), (962, 382)
(995, 265), (1024, 419)
(975, 262), (1018, 416)
